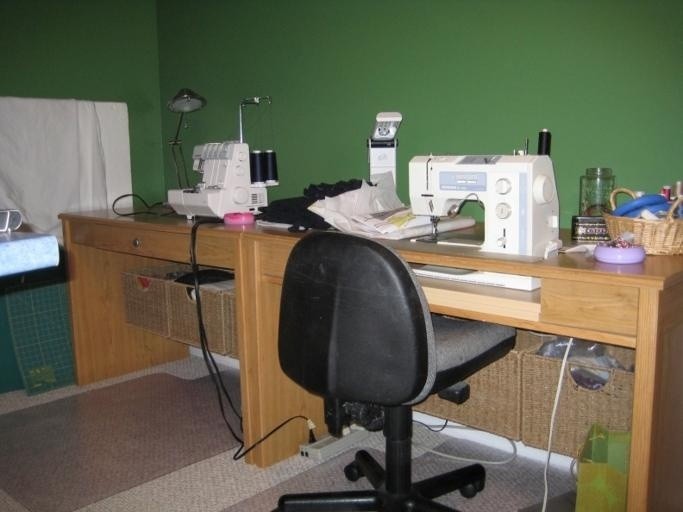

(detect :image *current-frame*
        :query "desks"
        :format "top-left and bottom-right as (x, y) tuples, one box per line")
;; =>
(59, 207), (253, 465)
(243, 216), (683, 510)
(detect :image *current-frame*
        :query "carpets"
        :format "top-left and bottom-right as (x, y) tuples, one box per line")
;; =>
(0, 370), (238, 512)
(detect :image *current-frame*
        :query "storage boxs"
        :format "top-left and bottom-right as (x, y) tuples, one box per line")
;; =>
(170, 281), (237, 356)
(124, 264), (187, 338)
(221, 287), (238, 358)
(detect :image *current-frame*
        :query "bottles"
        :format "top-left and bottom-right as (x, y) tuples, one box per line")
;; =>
(577, 167), (614, 218)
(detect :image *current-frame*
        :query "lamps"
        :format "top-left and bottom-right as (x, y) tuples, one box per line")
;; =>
(163, 85), (208, 189)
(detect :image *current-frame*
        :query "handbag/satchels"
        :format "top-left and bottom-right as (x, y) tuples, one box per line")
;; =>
(574, 424), (632, 512)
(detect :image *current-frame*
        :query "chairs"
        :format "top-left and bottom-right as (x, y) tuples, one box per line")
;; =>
(277, 232), (516, 510)
